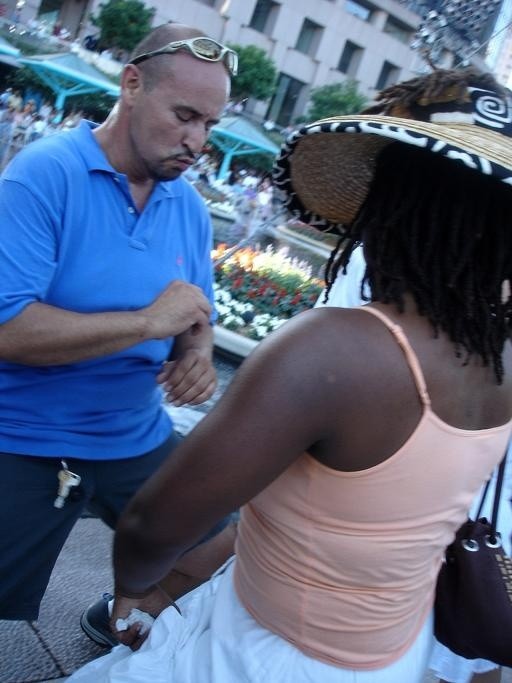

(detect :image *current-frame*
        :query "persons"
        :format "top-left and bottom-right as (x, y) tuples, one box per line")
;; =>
(0, 82), (89, 159)
(1, 21), (252, 656)
(55, 62), (510, 683)
(188, 140), (276, 210)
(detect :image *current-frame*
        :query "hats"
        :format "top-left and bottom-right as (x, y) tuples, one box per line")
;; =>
(270, 80), (512, 242)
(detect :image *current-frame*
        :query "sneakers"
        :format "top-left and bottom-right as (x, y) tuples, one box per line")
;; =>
(79, 594), (121, 648)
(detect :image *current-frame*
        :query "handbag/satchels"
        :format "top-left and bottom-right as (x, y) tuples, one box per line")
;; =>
(435, 518), (511, 667)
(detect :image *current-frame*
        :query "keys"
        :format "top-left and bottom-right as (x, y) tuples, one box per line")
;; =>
(53, 464), (82, 510)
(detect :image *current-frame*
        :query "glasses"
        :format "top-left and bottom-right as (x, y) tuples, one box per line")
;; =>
(127, 36), (240, 77)
